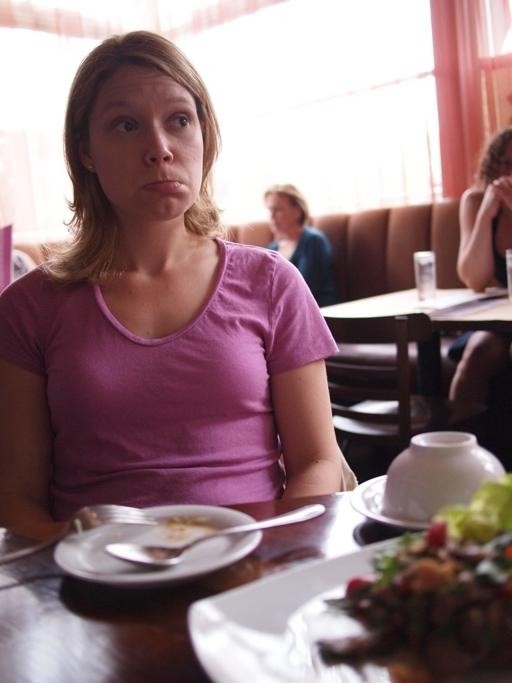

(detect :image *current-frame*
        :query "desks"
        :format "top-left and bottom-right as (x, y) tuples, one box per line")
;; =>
(319, 288), (509, 429)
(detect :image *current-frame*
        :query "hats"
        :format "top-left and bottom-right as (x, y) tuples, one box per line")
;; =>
(263, 182), (310, 219)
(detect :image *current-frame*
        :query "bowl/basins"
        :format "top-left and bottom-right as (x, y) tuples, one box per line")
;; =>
(379, 427), (508, 524)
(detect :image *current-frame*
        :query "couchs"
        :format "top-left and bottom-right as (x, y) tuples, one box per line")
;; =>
(217, 199), (464, 401)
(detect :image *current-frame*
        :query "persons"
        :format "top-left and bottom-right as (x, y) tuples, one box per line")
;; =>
(448, 129), (512, 413)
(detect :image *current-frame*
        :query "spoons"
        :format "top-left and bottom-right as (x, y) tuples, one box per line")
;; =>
(104, 499), (327, 569)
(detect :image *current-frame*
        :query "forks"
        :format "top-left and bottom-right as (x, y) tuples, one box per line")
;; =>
(0, 503), (158, 573)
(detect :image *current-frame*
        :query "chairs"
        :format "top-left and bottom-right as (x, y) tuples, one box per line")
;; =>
(320, 311), (487, 472)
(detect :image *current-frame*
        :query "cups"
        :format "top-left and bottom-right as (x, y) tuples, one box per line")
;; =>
(503, 247), (511, 303)
(411, 250), (437, 304)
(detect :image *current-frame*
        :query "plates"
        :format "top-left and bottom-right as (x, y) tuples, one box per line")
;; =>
(50, 501), (263, 591)
(352, 472), (440, 533)
(185, 532), (431, 683)
